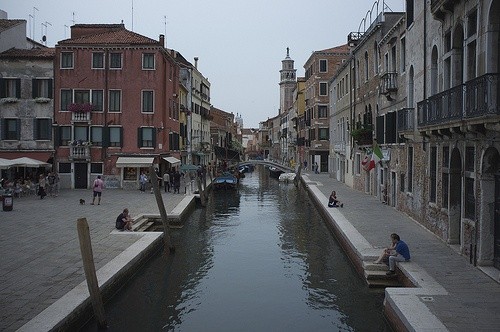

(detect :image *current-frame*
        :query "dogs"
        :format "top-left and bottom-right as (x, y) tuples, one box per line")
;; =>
(80, 198), (86, 205)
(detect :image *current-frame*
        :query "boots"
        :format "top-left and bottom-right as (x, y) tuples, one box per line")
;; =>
(150, 187), (153, 192)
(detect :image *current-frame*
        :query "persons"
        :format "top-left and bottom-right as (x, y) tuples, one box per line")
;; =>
(374, 233), (398, 264)
(0, 172), (60, 199)
(139, 162), (207, 194)
(314, 162), (319, 174)
(386, 234), (410, 275)
(328, 191), (340, 208)
(303, 160), (307, 169)
(115, 208), (134, 232)
(90, 175), (104, 205)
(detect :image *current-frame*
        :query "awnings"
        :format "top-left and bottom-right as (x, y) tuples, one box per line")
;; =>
(116, 157), (154, 167)
(164, 157), (181, 166)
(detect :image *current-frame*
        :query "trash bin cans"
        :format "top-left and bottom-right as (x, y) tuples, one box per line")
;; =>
(3, 194), (14, 211)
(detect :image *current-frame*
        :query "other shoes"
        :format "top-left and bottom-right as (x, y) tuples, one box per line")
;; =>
(373, 261), (381, 264)
(386, 270), (395, 275)
(90, 203), (94, 205)
(98, 203), (100, 205)
(341, 203), (343, 208)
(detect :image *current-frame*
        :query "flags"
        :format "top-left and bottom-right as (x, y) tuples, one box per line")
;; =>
(361, 144), (384, 172)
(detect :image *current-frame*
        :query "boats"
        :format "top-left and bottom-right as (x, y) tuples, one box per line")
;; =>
(231, 164), (254, 177)
(268, 166), (282, 177)
(212, 169), (237, 190)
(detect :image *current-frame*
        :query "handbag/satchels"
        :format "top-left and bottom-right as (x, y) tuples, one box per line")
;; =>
(95, 179), (98, 187)
(144, 178), (149, 182)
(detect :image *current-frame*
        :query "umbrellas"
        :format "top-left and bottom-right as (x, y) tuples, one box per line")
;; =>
(11, 157), (52, 177)
(0, 158), (12, 169)
